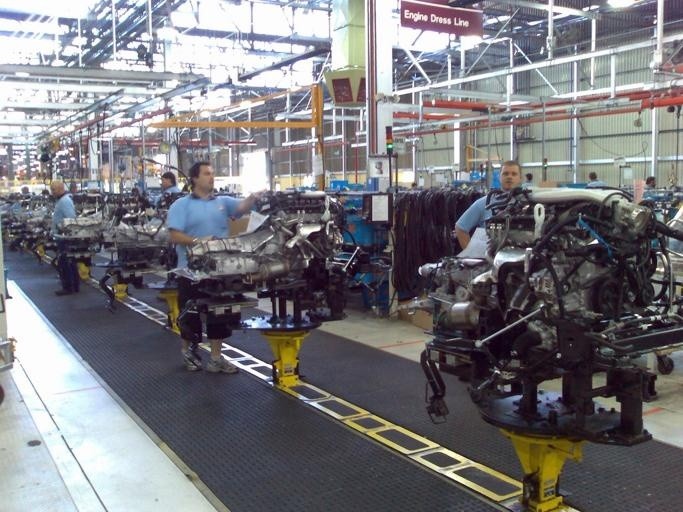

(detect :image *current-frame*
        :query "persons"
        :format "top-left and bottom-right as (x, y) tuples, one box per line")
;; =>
(522, 173), (536, 191)
(586, 171), (609, 187)
(0, 182), (79, 213)
(333, 184), (361, 252)
(165, 162), (267, 373)
(645, 176), (657, 189)
(149, 172), (179, 271)
(49, 180), (80, 296)
(455, 160), (524, 251)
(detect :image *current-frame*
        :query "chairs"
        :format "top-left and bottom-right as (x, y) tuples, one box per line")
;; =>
(54, 284), (81, 298)
(181, 352), (204, 372)
(206, 355), (239, 375)
